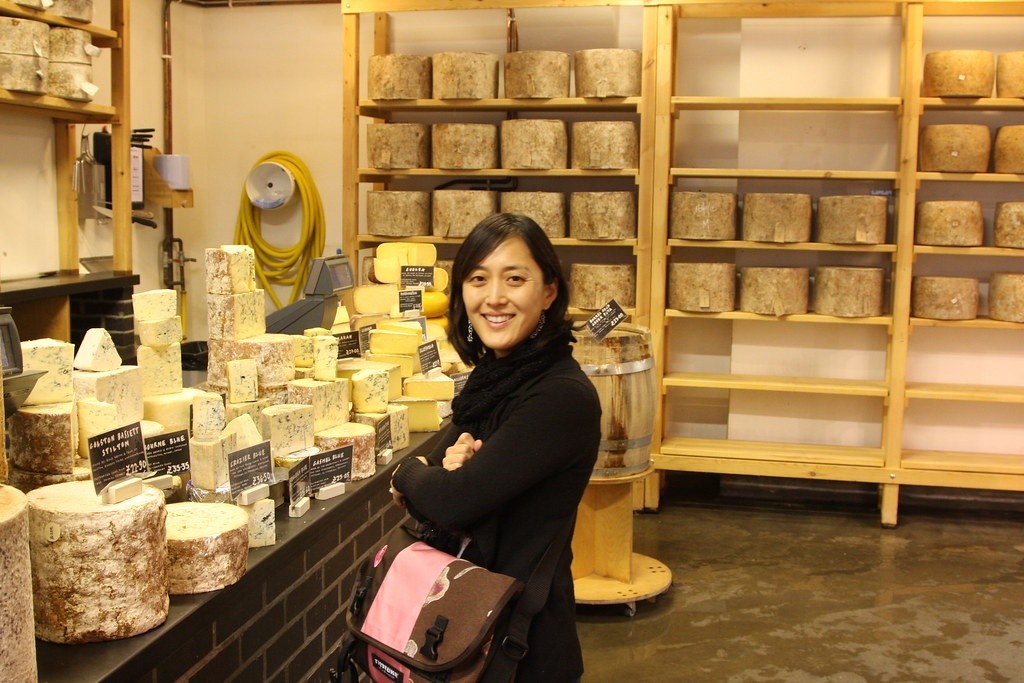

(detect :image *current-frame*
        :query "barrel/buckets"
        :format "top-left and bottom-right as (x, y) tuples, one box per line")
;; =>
(572, 326), (657, 478)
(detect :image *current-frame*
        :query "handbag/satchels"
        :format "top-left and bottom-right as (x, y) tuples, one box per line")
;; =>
(326, 522), (521, 683)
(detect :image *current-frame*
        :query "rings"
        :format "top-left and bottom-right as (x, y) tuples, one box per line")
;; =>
(389, 488), (393, 493)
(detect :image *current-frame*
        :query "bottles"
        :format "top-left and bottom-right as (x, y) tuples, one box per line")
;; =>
(75, 126), (155, 219)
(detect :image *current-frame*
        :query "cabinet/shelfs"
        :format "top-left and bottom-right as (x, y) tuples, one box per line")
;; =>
(341, 1), (658, 515)
(645, 0), (1024, 528)
(0, 0), (140, 342)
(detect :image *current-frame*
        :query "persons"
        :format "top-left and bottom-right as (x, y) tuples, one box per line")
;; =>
(391, 212), (602, 683)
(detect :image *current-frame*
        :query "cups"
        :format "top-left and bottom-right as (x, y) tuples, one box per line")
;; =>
(153, 155), (189, 190)
